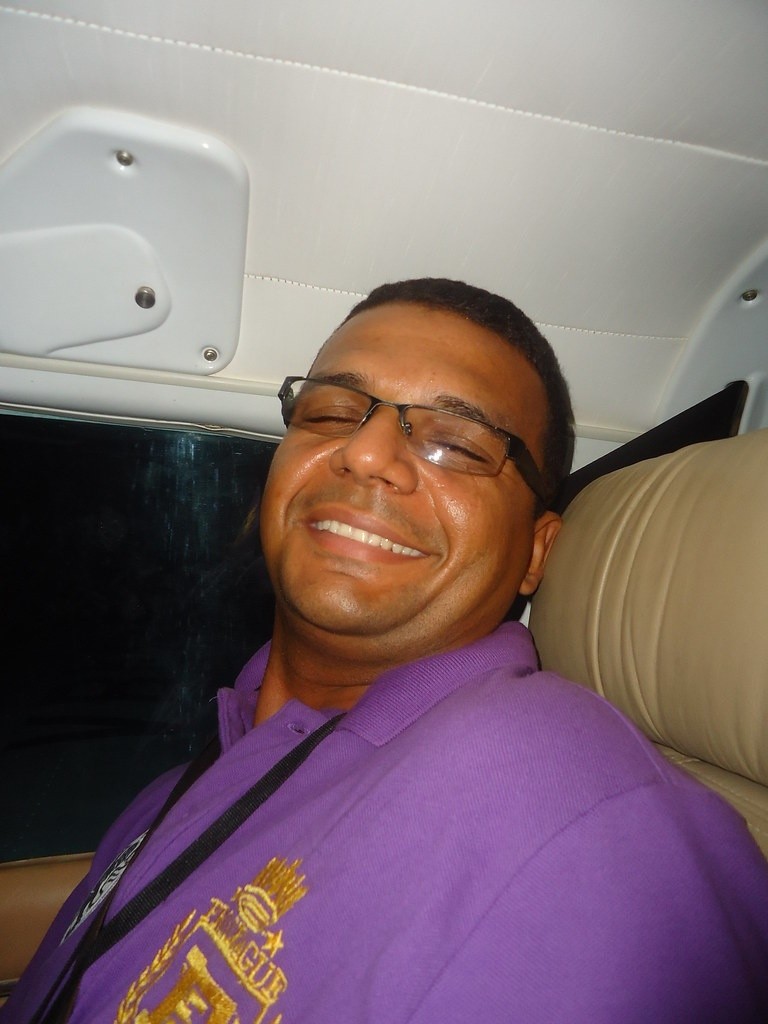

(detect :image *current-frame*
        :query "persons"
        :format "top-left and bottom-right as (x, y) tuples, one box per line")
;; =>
(0, 278), (768, 1024)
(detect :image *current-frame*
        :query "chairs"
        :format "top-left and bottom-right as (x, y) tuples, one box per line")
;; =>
(526, 423), (768, 863)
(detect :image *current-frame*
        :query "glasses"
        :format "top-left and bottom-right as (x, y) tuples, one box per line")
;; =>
(277, 376), (547, 502)
